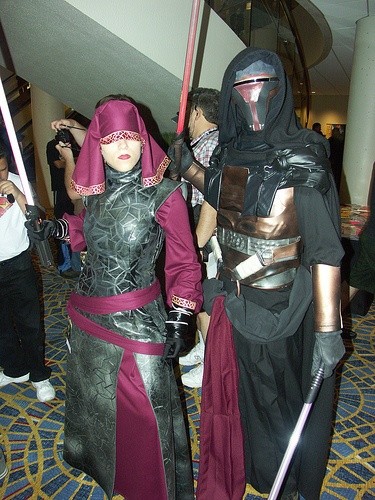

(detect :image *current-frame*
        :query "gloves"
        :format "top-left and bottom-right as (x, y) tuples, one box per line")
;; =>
(311, 330), (346, 380)
(25, 219), (57, 242)
(162, 310), (198, 365)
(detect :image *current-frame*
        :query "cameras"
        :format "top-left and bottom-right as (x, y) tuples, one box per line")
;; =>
(58, 128), (70, 147)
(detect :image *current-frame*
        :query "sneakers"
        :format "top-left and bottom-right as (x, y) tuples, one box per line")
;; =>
(31, 378), (55, 402)
(0, 369), (30, 387)
(179, 329), (205, 365)
(59, 268), (81, 279)
(180, 361), (203, 388)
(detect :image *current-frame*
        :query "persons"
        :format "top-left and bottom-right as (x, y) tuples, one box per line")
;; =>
(166, 47), (347, 500)
(0, 147), (57, 403)
(25, 100), (204, 500)
(312, 123), (326, 138)
(179, 88), (221, 389)
(50, 94), (136, 201)
(46, 129), (78, 219)
(329, 127), (344, 195)
(342, 162), (374, 309)
